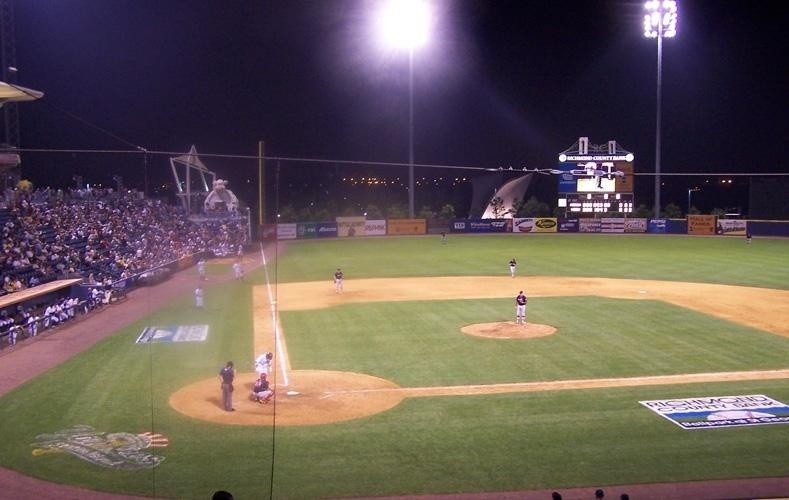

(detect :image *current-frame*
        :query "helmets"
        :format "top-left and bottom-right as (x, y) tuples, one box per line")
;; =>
(266, 352), (273, 360)
(261, 373), (267, 381)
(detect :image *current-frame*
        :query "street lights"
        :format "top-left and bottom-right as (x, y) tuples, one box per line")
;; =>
(639, 0), (680, 223)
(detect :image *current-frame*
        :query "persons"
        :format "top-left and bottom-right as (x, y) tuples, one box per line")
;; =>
(0, 180), (251, 350)
(333, 266), (344, 295)
(508, 257), (516, 277)
(253, 372), (273, 404)
(254, 351), (273, 386)
(745, 229), (752, 247)
(439, 232), (448, 240)
(217, 360), (236, 411)
(515, 290), (527, 325)
